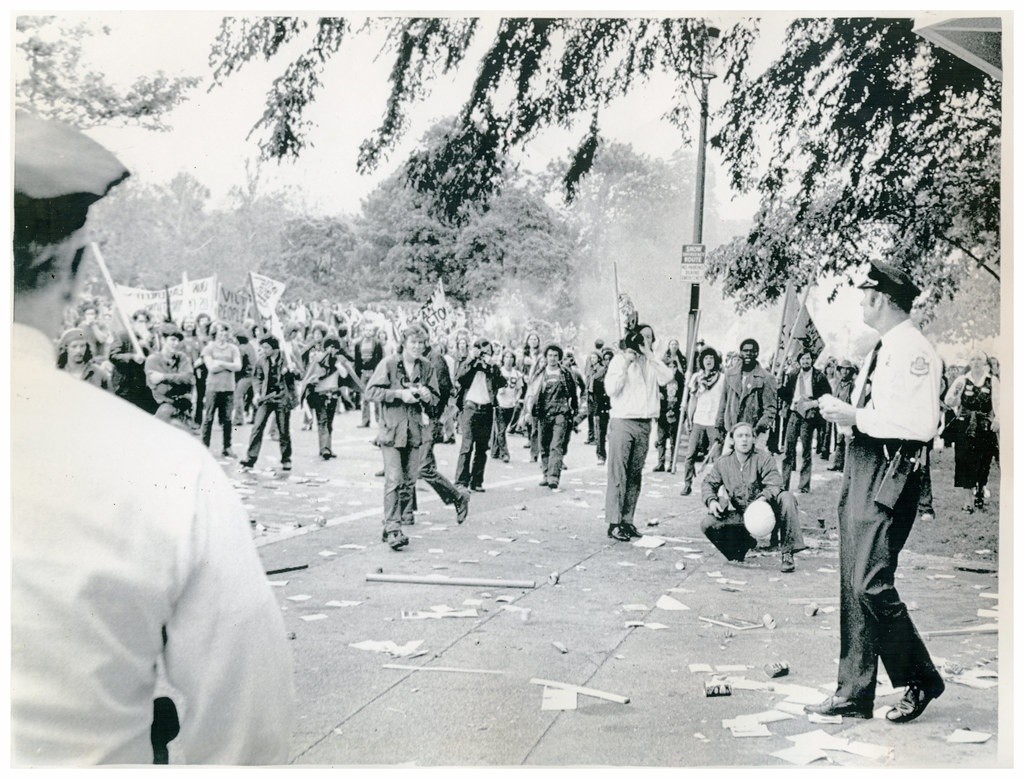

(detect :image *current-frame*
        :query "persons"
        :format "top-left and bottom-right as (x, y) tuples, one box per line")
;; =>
(804, 257), (945, 723)
(59, 299), (1001, 572)
(12, 110), (294, 766)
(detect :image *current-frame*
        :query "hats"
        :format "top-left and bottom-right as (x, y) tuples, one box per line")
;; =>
(57, 328), (85, 350)
(14, 109), (132, 242)
(162, 326), (184, 342)
(857, 258), (921, 302)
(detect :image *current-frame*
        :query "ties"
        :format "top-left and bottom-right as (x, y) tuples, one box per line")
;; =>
(851, 340), (882, 437)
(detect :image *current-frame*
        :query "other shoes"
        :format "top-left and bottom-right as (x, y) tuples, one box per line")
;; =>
(240, 460), (254, 468)
(382, 530), (409, 549)
(607, 521), (642, 541)
(375, 471), (385, 476)
(539, 479), (558, 488)
(781, 552), (795, 572)
(282, 461), (291, 470)
(596, 445), (992, 522)
(223, 448), (239, 459)
(455, 485), (472, 494)
(401, 513), (414, 524)
(475, 485), (485, 492)
(454, 493), (470, 524)
(319, 451), (337, 460)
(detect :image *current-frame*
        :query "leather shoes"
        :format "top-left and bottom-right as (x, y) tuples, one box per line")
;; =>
(802, 696), (874, 719)
(885, 677), (945, 722)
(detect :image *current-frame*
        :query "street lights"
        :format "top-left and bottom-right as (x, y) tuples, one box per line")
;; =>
(676, 27), (720, 463)
